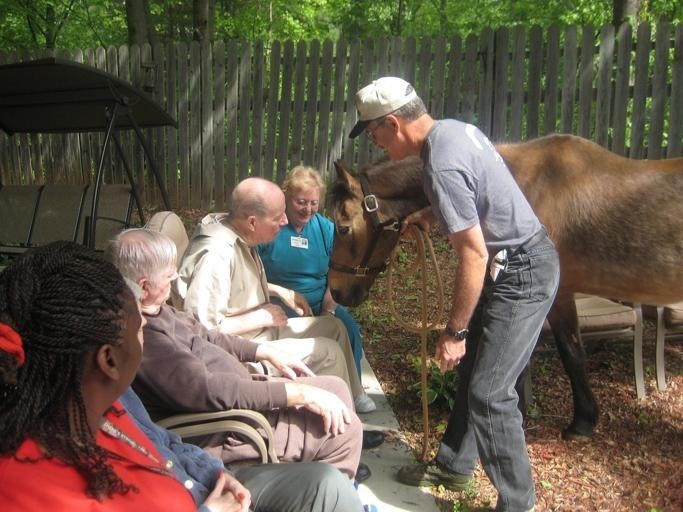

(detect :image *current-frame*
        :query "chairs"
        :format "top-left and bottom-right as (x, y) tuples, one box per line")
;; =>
(158, 409), (280, 466)
(0, 182), (45, 255)
(520, 291), (647, 413)
(26, 182), (91, 260)
(623, 294), (683, 391)
(74, 183), (139, 256)
(164, 418), (268, 467)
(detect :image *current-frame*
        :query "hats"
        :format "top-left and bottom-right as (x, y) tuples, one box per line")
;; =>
(348, 76), (415, 139)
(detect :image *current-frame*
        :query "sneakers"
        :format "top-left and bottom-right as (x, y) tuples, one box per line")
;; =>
(354, 392), (376, 413)
(398, 457), (470, 491)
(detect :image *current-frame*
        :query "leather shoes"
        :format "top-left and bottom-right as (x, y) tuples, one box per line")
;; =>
(357, 462), (371, 481)
(362, 430), (384, 448)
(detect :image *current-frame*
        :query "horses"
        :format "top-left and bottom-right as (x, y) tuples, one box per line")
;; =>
(327, 133), (683, 444)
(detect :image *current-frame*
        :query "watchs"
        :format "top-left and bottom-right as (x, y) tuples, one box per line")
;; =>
(445, 325), (469, 340)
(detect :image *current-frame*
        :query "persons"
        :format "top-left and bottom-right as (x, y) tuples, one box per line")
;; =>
(117, 275), (364, 512)
(0, 241), (196, 511)
(105, 228), (363, 485)
(348, 76), (561, 512)
(171, 178), (384, 482)
(255, 165), (377, 413)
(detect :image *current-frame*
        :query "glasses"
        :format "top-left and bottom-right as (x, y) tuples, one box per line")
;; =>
(363, 117), (384, 138)
